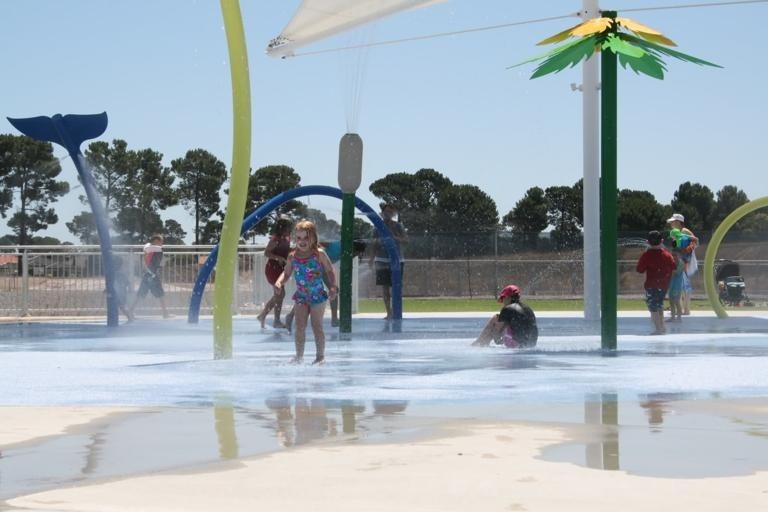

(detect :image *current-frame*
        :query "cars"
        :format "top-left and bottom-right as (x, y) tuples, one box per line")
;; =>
(0, 253), (125, 277)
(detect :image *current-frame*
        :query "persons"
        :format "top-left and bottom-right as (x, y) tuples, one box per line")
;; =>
(368, 204), (408, 320)
(470, 285), (539, 349)
(285, 240), (368, 331)
(132, 234), (175, 320)
(256, 218), (294, 330)
(273, 219), (339, 368)
(102, 253), (136, 328)
(635, 212), (700, 335)
(279, 402), (334, 448)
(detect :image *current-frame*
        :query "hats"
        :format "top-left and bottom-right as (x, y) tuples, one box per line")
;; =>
(666, 214), (685, 223)
(497, 285), (519, 303)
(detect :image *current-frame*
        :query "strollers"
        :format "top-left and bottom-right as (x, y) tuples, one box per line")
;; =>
(713, 259), (754, 307)
